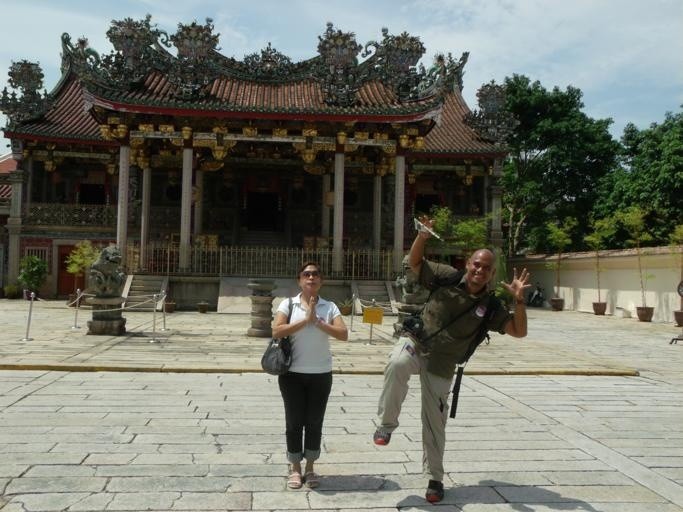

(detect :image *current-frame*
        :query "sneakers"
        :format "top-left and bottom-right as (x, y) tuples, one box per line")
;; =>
(373, 425), (391, 445)
(425, 479), (444, 503)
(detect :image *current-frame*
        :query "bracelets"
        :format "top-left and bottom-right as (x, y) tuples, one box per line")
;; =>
(315, 318), (324, 326)
(513, 299), (526, 305)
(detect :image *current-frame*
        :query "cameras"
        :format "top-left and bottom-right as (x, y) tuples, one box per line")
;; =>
(403, 317), (423, 335)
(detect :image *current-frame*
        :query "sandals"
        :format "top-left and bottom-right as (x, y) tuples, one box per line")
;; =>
(286, 469), (321, 488)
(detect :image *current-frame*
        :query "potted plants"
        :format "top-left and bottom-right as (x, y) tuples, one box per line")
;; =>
(545, 215), (578, 311)
(619, 208), (654, 322)
(18, 255), (48, 300)
(64, 239), (99, 307)
(584, 217), (618, 315)
(669, 225), (683, 326)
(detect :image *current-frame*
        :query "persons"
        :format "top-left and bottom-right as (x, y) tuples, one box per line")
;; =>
(271, 262), (348, 489)
(374, 214), (533, 502)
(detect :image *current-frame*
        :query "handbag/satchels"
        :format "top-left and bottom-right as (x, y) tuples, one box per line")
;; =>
(262, 296), (294, 375)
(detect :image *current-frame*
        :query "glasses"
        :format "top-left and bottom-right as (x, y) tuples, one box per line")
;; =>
(302, 270), (320, 279)
